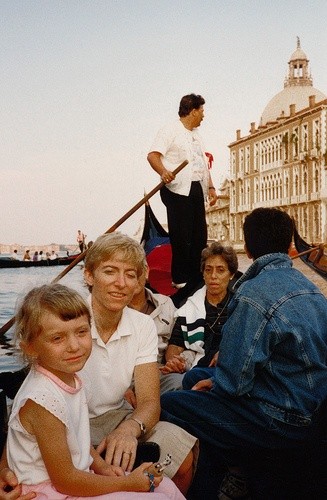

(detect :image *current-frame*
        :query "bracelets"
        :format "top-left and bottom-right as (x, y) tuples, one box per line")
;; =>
(209, 187), (215, 189)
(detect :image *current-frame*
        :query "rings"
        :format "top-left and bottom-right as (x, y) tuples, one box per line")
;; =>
(123, 451), (131, 454)
(166, 179), (168, 182)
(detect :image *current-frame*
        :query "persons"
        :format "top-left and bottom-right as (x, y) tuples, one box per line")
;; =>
(7, 285), (186, 500)
(147, 94), (218, 293)
(160, 209), (327, 500)
(33, 251), (57, 261)
(160, 245), (238, 374)
(77, 230), (85, 254)
(125, 256), (183, 406)
(0, 232), (198, 500)
(24, 250), (30, 261)
(11, 250), (18, 259)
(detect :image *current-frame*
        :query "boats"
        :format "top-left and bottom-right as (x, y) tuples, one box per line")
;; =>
(0, 252), (83, 268)
(293, 226), (327, 281)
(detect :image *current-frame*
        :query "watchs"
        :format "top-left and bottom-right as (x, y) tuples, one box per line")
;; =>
(129, 417), (146, 439)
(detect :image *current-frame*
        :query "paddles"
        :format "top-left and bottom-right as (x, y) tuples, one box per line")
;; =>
(290, 243), (327, 260)
(72, 235), (88, 255)
(0, 159), (191, 337)
